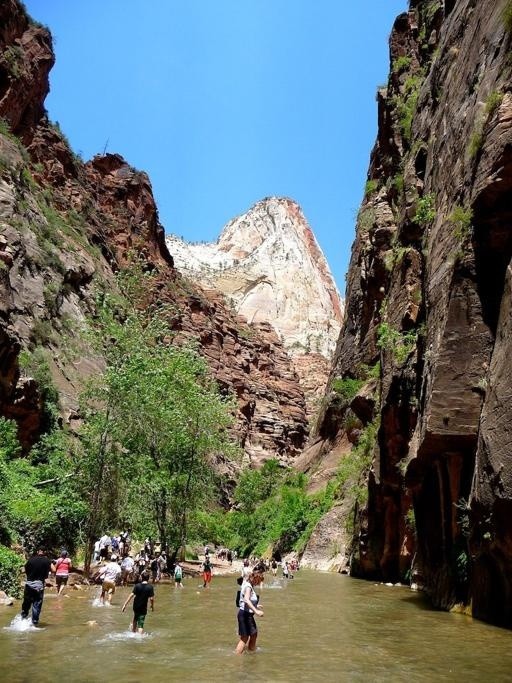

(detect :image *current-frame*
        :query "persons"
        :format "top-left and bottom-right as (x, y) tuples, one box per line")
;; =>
(54, 550), (72, 595)
(21, 545), (57, 624)
(122, 572), (155, 634)
(94, 531), (168, 604)
(174, 562), (183, 589)
(200, 545), (299, 587)
(235, 571), (264, 654)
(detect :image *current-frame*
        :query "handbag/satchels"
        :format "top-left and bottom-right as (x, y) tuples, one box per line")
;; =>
(175, 574), (181, 580)
(236, 591), (259, 607)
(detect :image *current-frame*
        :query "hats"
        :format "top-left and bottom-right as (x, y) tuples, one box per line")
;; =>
(60, 550), (68, 557)
(244, 559), (249, 565)
(111, 555), (117, 560)
(253, 565), (265, 571)
(142, 573), (149, 578)
